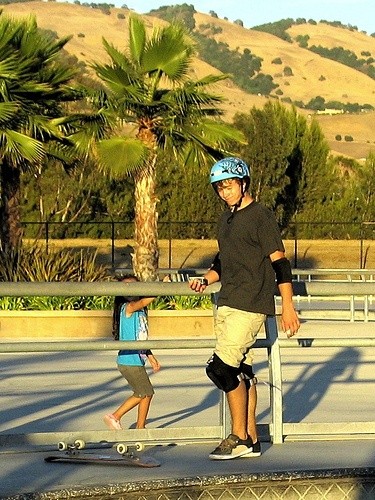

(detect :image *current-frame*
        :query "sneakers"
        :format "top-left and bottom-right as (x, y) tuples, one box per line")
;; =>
(209, 434), (253, 460)
(240, 440), (262, 457)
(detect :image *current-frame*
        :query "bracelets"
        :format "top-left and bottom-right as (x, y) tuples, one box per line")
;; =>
(204, 279), (208, 287)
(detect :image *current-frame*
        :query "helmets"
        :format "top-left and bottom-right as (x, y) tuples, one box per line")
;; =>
(209, 157), (251, 185)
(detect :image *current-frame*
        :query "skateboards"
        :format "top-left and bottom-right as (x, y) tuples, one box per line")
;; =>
(44, 439), (161, 467)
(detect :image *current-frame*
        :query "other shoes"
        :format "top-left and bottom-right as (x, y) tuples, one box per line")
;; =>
(102, 414), (122, 430)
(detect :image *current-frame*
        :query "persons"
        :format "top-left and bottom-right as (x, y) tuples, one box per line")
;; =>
(103, 275), (159, 430)
(190, 157), (300, 461)
